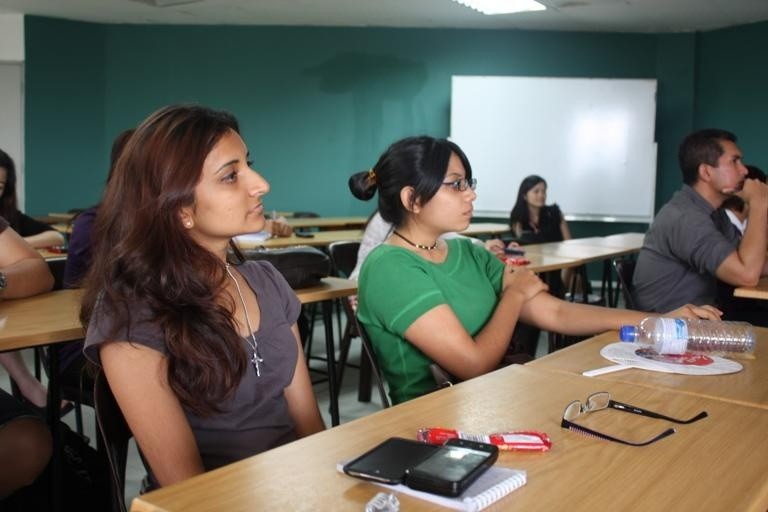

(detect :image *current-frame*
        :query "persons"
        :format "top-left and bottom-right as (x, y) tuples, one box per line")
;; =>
(44, 128), (161, 504)
(508, 173), (572, 355)
(710, 163), (767, 248)
(347, 203), (518, 313)
(1, 148), (76, 417)
(79, 102), (325, 496)
(0, 211), (55, 506)
(630, 126), (767, 315)
(232, 215), (293, 245)
(347, 134), (724, 406)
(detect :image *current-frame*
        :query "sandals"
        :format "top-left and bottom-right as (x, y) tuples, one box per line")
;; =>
(20, 393), (74, 421)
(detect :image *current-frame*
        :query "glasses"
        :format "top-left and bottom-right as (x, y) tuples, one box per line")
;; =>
(560, 390), (709, 448)
(442, 178), (478, 192)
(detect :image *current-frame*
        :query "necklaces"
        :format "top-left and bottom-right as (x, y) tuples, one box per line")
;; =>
(216, 260), (263, 380)
(392, 229), (439, 253)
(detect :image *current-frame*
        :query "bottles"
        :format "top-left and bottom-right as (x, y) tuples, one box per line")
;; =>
(617, 314), (757, 359)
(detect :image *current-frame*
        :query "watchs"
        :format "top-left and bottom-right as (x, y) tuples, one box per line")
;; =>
(1, 270), (8, 292)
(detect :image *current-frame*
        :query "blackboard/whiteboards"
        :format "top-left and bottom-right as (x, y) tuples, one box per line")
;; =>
(446, 74), (658, 224)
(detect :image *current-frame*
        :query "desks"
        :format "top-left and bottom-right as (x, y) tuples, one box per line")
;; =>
(1, 212), (767, 512)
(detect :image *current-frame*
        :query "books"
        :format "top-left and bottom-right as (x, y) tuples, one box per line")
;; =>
(334, 451), (528, 512)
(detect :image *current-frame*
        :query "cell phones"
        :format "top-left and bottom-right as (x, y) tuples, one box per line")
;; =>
(343, 436), (439, 486)
(296, 232), (314, 237)
(405, 438), (499, 498)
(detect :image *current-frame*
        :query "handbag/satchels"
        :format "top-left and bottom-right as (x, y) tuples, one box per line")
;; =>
(427, 335), (534, 391)
